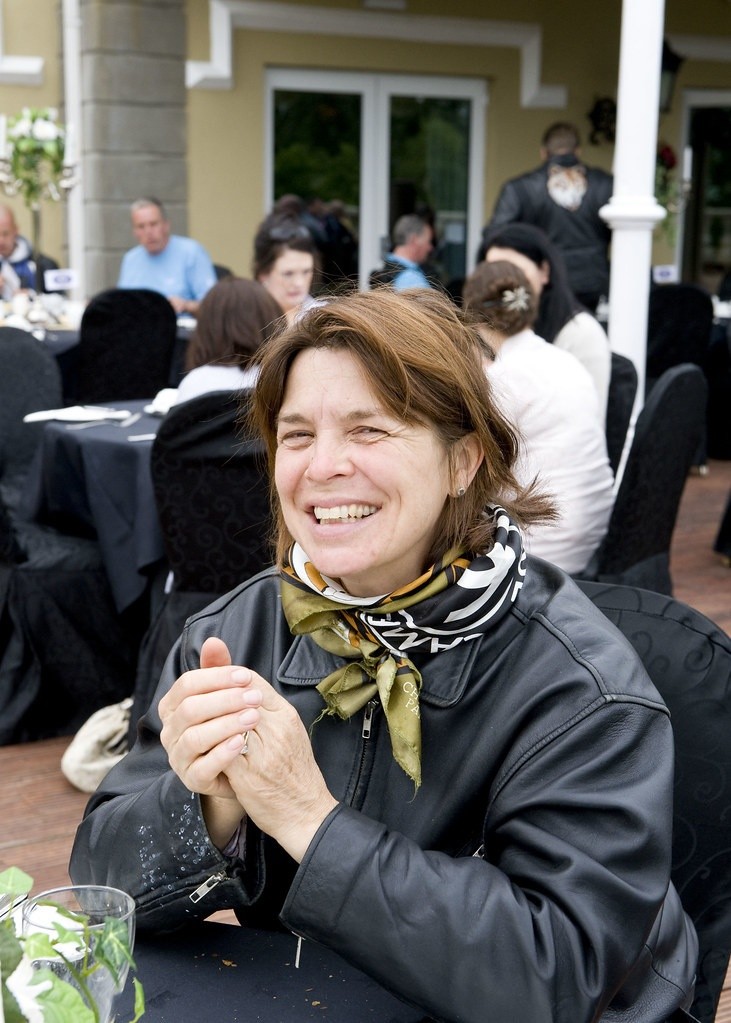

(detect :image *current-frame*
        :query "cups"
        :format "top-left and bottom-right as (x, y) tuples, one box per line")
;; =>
(21, 885), (135, 1023)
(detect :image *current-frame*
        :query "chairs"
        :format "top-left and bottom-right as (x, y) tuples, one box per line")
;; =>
(0, 256), (728, 1023)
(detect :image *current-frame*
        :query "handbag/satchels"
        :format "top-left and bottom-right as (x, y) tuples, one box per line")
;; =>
(59, 693), (134, 791)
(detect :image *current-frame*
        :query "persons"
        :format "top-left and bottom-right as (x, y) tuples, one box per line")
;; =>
(459, 260), (616, 577)
(476, 120), (616, 322)
(250, 212), (330, 334)
(67, 282), (707, 1023)
(167, 275), (289, 408)
(370, 214), (435, 292)
(115, 195), (219, 320)
(0, 201), (67, 301)
(270, 193), (362, 286)
(473, 224), (612, 446)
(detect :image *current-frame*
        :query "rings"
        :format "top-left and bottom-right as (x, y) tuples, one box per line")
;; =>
(239, 729), (250, 755)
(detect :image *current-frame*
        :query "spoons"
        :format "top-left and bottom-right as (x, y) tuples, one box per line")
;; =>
(65, 413), (142, 430)
